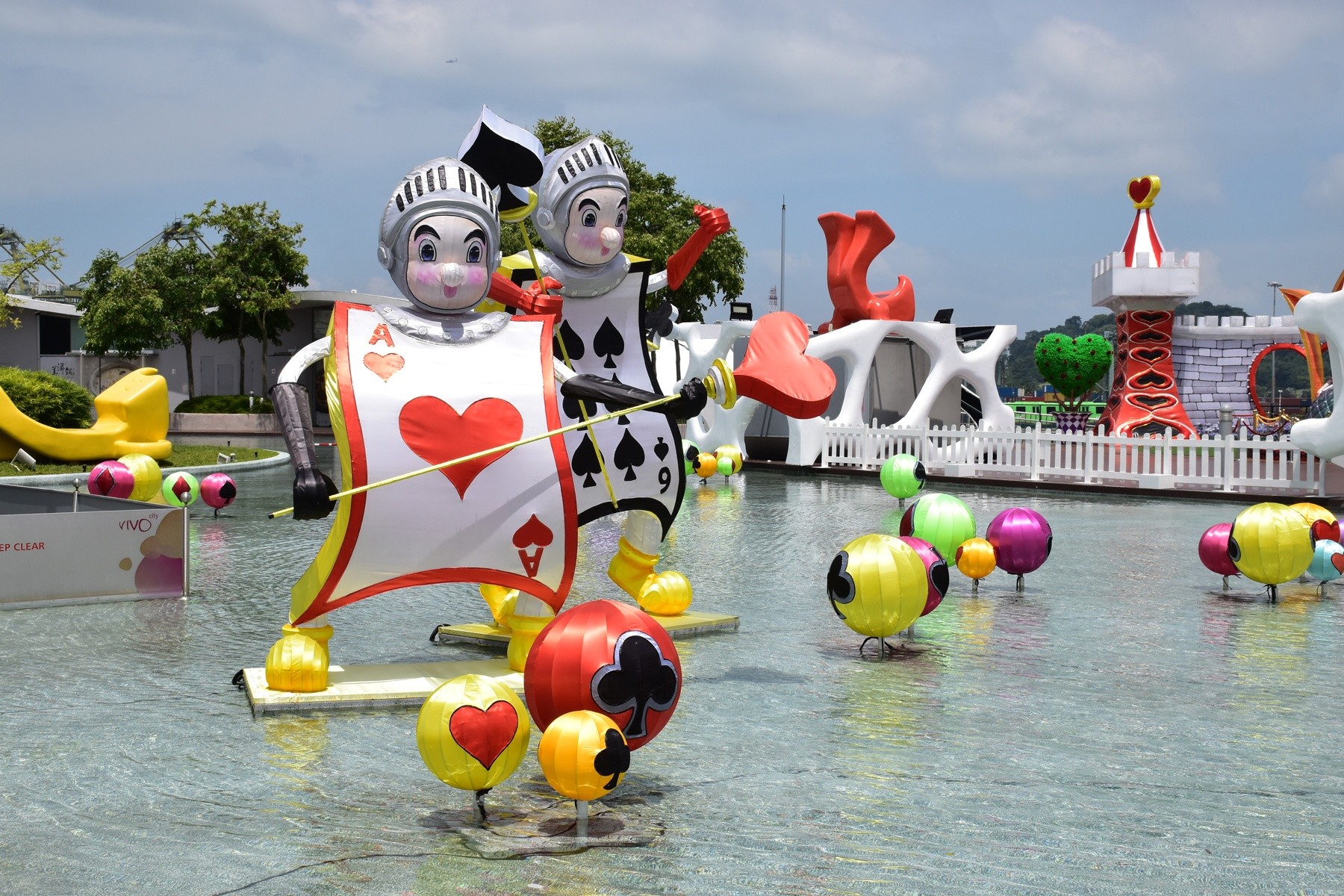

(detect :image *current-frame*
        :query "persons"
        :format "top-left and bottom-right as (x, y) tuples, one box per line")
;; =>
(478, 137), (727, 625)
(264, 159), (708, 693)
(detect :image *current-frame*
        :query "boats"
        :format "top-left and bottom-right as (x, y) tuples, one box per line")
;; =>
(1004, 402), (1107, 427)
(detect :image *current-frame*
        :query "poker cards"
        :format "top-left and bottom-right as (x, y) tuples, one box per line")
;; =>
(288, 254), (687, 628)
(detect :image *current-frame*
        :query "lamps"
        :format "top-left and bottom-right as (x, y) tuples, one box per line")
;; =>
(216, 453), (231, 464)
(10, 448), (36, 472)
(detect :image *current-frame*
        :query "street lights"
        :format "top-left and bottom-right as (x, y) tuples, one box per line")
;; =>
(1267, 281), (1283, 403)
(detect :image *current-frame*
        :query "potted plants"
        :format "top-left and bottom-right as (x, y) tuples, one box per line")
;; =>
(1034, 332), (1114, 443)
